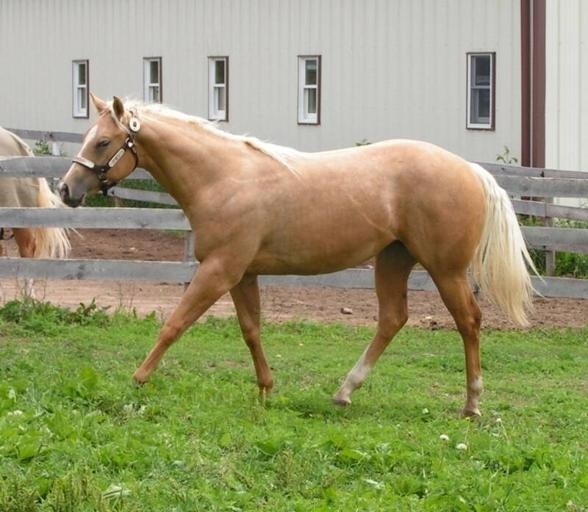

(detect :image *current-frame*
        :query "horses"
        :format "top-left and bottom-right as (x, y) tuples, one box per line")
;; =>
(0, 121), (84, 323)
(56, 90), (547, 422)
